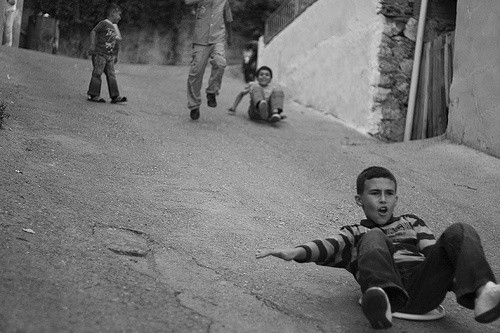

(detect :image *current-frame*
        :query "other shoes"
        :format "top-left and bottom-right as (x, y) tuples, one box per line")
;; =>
(259, 100), (269, 120)
(474, 281), (500, 324)
(88, 95), (106, 103)
(207, 93), (216, 107)
(269, 112), (281, 123)
(190, 108), (200, 120)
(361, 287), (393, 330)
(110, 96), (127, 103)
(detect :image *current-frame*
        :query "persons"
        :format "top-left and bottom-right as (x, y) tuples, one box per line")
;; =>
(186, 0), (233, 119)
(229, 66), (284, 122)
(256, 167), (500, 328)
(87, 4), (127, 102)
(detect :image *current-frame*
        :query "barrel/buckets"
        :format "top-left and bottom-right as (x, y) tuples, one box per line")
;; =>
(26, 15), (56, 54)
